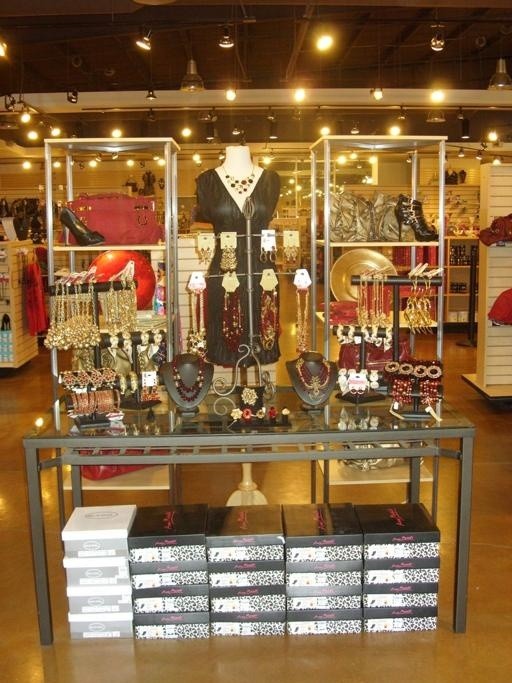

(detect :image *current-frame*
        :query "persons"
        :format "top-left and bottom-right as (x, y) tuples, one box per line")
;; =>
(141, 169), (157, 196)
(194, 144), (283, 365)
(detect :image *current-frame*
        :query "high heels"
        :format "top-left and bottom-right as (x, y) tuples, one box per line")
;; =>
(396, 192), (438, 242)
(61, 205), (105, 246)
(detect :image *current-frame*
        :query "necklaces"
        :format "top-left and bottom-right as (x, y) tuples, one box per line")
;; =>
(223, 161), (255, 195)
(43, 243), (442, 426)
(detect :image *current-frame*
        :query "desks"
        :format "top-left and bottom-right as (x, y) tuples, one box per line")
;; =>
(21, 391), (476, 649)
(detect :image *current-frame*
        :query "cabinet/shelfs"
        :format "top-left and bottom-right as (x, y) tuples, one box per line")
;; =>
(448, 227), (478, 327)
(307, 135), (454, 403)
(45, 137), (177, 434)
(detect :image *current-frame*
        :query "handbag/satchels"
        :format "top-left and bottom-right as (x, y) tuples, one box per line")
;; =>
(328, 191), (375, 242)
(365, 192), (415, 241)
